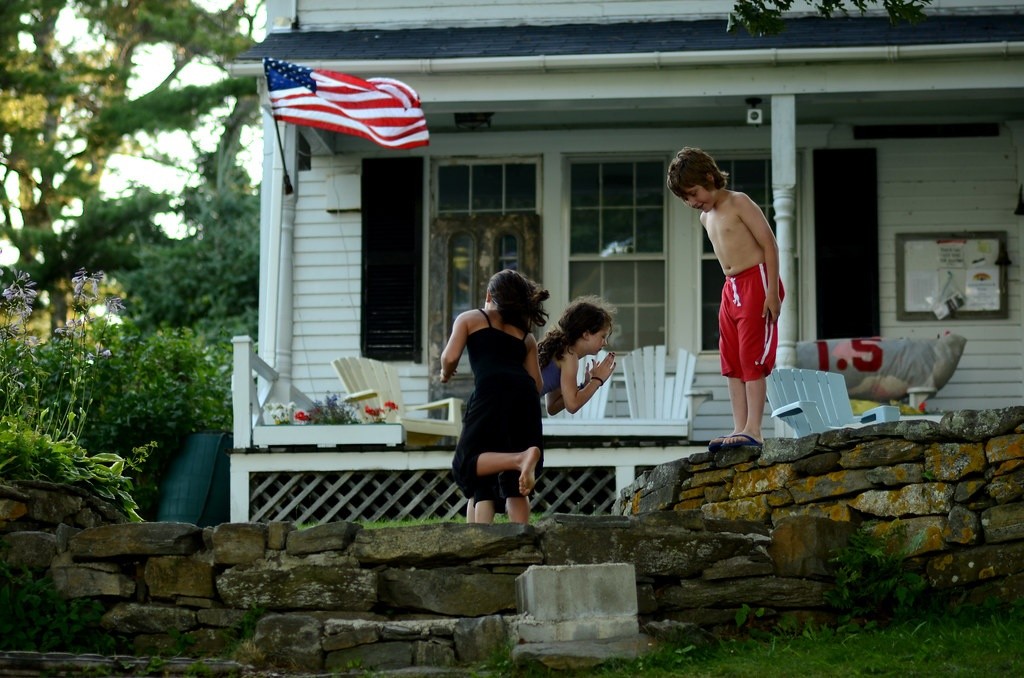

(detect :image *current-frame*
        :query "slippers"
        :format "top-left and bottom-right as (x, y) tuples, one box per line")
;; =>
(720, 434), (762, 447)
(709, 436), (727, 451)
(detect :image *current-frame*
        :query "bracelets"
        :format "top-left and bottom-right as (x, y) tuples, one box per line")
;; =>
(591, 376), (603, 386)
(578, 383), (586, 390)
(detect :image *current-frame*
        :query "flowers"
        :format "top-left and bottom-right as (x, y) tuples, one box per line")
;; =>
(262, 390), (398, 426)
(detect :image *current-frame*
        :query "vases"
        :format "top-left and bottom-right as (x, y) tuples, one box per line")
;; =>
(253, 423), (406, 451)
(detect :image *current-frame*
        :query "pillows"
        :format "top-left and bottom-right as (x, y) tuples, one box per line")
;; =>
(796, 335), (968, 402)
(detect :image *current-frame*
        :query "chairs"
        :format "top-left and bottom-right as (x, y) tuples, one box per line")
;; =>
(765, 369), (902, 438)
(540, 344), (714, 446)
(331, 356), (464, 448)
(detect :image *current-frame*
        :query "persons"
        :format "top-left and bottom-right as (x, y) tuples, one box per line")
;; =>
(438, 269), (550, 524)
(666, 146), (785, 449)
(463, 293), (616, 523)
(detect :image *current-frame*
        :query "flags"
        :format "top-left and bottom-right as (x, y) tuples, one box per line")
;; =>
(260, 55), (430, 150)
